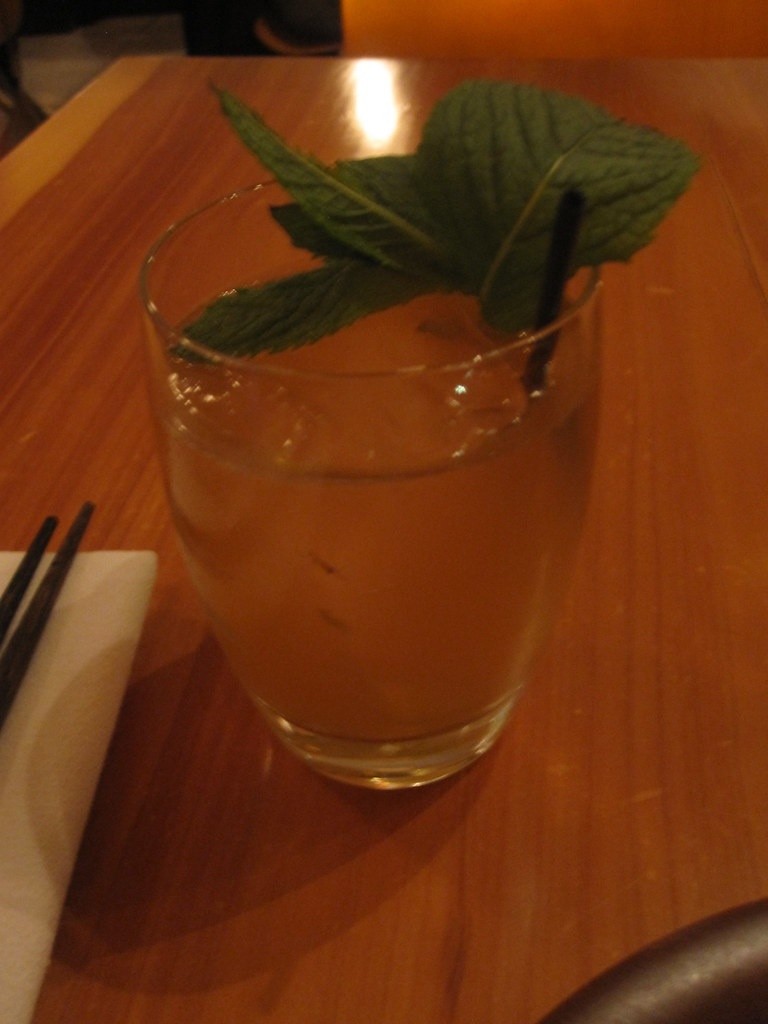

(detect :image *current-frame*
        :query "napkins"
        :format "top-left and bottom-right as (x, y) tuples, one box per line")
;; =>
(0, 548), (159, 1024)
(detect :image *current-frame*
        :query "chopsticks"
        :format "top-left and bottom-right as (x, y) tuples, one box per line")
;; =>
(0, 500), (96, 730)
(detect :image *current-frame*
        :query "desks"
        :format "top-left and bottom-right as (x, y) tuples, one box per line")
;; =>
(0, 57), (768, 1024)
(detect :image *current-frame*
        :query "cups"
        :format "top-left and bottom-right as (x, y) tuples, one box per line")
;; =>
(138, 178), (603, 790)
